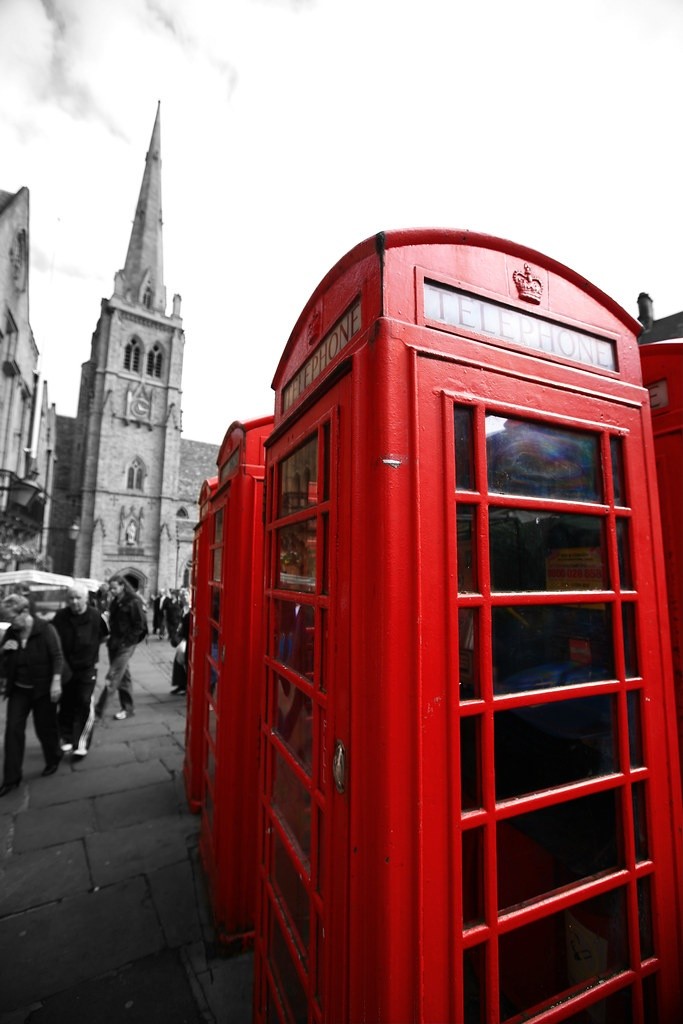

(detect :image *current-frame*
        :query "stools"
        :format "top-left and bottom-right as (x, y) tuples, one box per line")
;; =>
(463, 796), (557, 1010)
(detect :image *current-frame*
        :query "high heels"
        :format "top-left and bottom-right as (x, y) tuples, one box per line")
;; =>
(0, 777), (22, 796)
(41, 750), (64, 776)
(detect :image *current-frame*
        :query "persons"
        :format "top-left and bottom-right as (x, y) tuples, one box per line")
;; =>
(1, 583), (192, 759)
(94, 575), (148, 723)
(0, 593), (73, 795)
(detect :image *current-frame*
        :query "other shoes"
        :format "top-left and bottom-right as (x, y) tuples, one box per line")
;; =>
(72, 747), (88, 756)
(60, 742), (74, 752)
(171, 687), (187, 695)
(114, 708), (128, 719)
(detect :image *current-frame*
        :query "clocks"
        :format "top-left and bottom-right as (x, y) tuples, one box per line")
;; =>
(131, 399), (150, 418)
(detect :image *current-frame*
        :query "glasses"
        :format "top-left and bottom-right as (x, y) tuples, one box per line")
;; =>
(5, 614), (21, 621)
